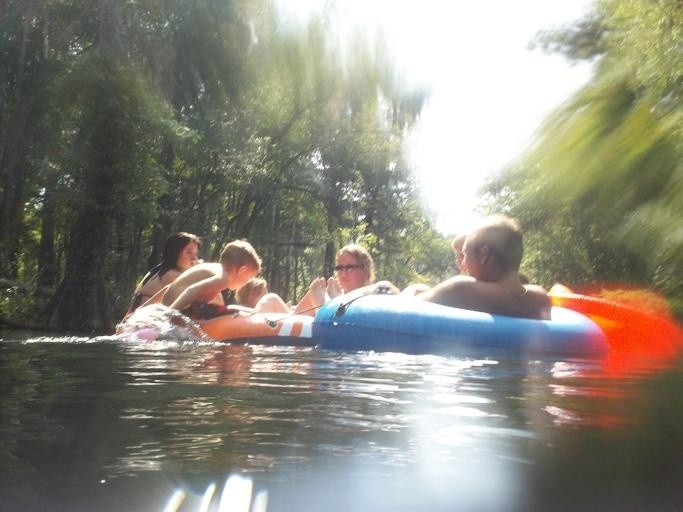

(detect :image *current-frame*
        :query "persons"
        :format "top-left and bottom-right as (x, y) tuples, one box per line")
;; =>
(125, 232), (290, 323)
(309, 244), (375, 316)
(403, 222), (552, 323)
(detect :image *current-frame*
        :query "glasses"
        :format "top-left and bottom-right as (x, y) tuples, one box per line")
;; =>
(334, 264), (364, 272)
